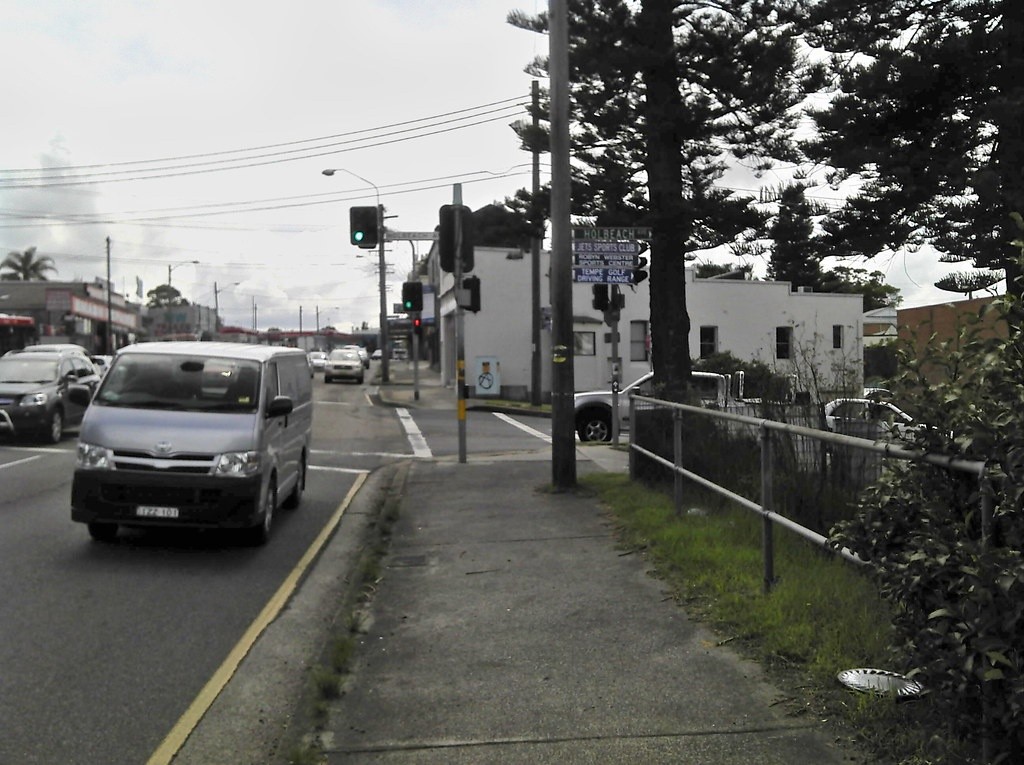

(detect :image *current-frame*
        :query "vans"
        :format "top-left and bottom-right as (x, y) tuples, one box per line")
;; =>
(69, 340), (314, 545)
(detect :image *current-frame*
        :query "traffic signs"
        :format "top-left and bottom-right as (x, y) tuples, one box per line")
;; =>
(574, 239), (639, 257)
(572, 252), (639, 270)
(573, 267), (636, 283)
(572, 224), (654, 241)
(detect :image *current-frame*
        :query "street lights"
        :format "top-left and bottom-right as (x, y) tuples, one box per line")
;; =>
(168, 260), (203, 334)
(322, 167), (382, 205)
(214, 281), (242, 341)
(316, 304), (342, 351)
(321, 168), (390, 382)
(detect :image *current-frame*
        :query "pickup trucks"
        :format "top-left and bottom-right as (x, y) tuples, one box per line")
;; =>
(574, 366), (800, 442)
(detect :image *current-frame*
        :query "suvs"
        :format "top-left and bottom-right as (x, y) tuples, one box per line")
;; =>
(819, 398), (940, 444)
(22, 343), (100, 376)
(0, 347), (104, 446)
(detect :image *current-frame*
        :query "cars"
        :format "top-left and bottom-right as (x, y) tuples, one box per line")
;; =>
(309, 352), (329, 368)
(339, 343), (370, 371)
(323, 349), (363, 385)
(864, 388), (896, 402)
(92, 354), (113, 376)
(369, 348), (382, 361)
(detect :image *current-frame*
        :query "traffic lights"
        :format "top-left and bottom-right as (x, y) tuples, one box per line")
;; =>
(349, 206), (378, 245)
(592, 284), (610, 312)
(462, 275), (481, 314)
(413, 317), (423, 334)
(402, 280), (424, 311)
(634, 241), (649, 285)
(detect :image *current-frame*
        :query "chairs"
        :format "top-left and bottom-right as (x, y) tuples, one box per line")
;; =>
(222, 366), (256, 402)
(141, 365), (175, 397)
